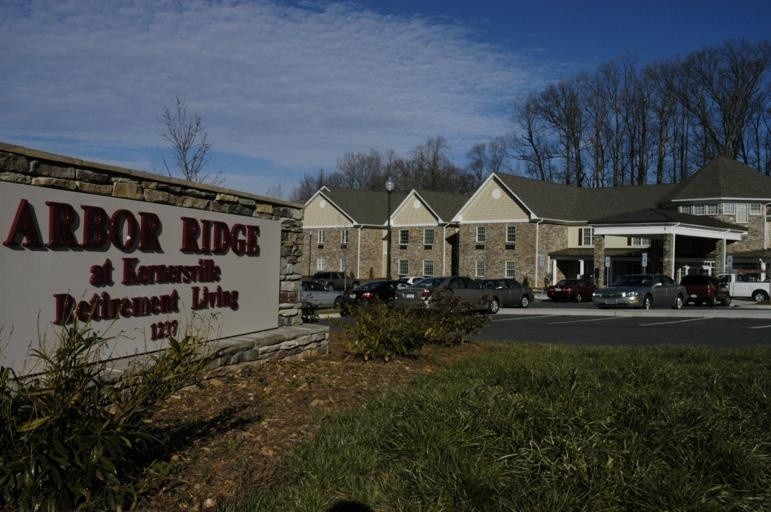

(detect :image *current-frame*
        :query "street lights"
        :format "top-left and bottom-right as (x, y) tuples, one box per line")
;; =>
(385, 176), (395, 280)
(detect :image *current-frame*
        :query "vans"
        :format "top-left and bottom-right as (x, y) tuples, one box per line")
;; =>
(313, 270), (360, 290)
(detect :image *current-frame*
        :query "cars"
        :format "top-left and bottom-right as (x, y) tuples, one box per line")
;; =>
(301, 276), (347, 309)
(344, 274), (534, 314)
(592, 273), (689, 310)
(678, 276), (731, 306)
(548, 279), (597, 302)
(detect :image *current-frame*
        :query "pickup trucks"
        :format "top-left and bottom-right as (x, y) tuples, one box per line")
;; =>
(716, 273), (770, 302)
